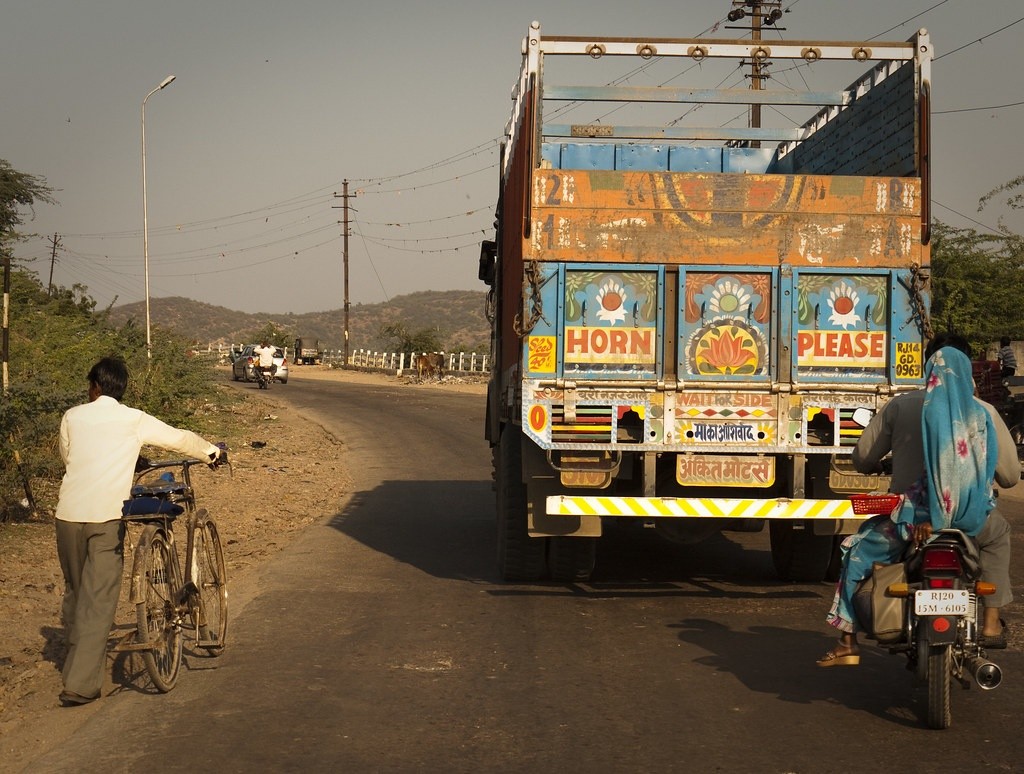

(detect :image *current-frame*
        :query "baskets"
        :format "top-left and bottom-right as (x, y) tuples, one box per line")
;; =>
(849, 492), (901, 514)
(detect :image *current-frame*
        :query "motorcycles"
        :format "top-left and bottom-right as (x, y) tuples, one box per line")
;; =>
(251, 358), (277, 391)
(845, 406), (1003, 732)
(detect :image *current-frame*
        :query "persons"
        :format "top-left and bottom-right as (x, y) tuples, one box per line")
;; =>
(997, 336), (1017, 376)
(55, 358), (221, 703)
(815, 335), (1022, 667)
(253, 341), (277, 381)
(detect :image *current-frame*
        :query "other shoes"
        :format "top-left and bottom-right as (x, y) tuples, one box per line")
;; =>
(60, 692), (102, 704)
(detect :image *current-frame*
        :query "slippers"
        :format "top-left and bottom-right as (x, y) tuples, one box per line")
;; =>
(818, 651), (859, 668)
(981, 619), (1012, 646)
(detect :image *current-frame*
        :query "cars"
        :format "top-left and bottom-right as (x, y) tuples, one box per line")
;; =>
(231, 344), (288, 383)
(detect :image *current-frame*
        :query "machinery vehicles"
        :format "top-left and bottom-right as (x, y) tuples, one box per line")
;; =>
(967, 348), (1024, 480)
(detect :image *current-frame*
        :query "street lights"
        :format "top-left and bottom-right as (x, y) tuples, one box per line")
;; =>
(140, 72), (176, 360)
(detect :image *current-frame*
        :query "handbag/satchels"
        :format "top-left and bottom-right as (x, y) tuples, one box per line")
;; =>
(852, 560), (909, 641)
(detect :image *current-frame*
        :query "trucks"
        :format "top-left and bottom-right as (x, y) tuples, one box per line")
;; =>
(476, 22), (937, 587)
(293, 337), (321, 364)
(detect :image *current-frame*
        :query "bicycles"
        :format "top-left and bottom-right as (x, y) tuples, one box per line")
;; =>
(107, 443), (230, 691)
